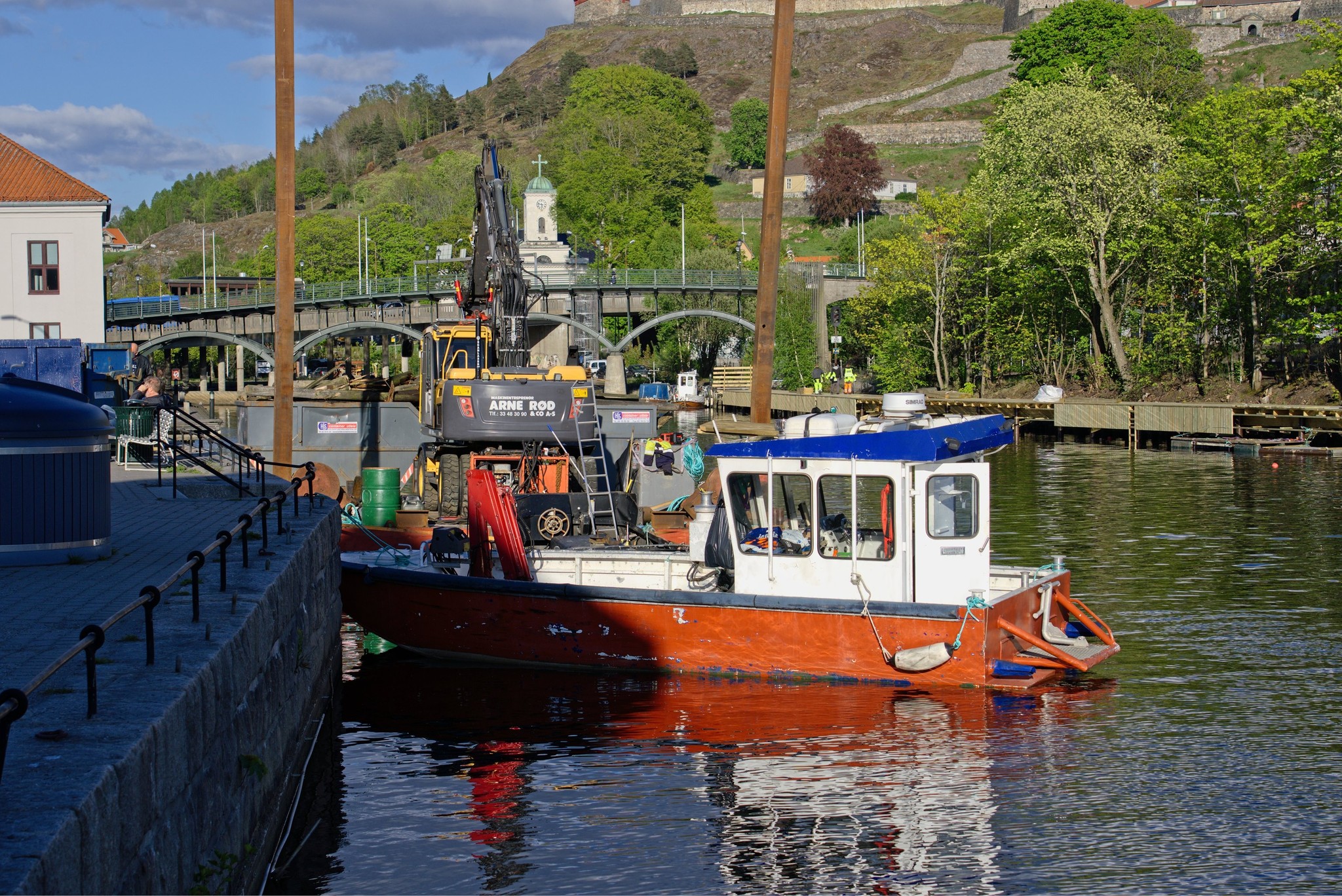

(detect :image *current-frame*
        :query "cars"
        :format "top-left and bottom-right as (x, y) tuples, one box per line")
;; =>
(336, 335), (400, 346)
(626, 364), (652, 377)
(369, 302), (409, 320)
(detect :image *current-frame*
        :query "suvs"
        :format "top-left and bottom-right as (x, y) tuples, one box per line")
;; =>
(307, 358), (338, 380)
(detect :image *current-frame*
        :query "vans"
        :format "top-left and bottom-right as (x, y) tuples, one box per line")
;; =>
(585, 359), (607, 380)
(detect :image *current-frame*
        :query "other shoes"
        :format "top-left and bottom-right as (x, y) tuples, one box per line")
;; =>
(831, 392), (833, 395)
(837, 393), (839, 394)
(844, 392), (847, 394)
(849, 392), (853, 394)
(819, 390), (821, 394)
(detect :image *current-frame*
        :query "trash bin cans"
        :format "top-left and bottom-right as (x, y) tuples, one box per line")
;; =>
(0, 377), (112, 565)
(110, 405), (158, 463)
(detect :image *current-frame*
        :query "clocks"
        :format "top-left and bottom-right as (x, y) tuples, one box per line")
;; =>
(537, 200), (546, 210)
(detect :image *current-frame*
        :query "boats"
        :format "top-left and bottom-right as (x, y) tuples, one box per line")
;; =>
(293, 139), (1120, 694)
(1170, 437), (1311, 447)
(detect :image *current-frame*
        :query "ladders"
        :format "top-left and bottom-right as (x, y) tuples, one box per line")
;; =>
(571, 385), (620, 541)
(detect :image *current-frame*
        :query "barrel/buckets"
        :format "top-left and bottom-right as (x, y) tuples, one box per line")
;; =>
(361, 467), (400, 528)
(401, 495), (425, 510)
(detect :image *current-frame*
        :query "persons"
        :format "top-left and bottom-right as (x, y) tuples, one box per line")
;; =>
(810, 363), (825, 395)
(829, 363), (842, 395)
(106, 377), (171, 439)
(843, 363), (855, 395)
(131, 343), (153, 397)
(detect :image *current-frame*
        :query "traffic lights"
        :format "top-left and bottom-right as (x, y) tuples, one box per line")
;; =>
(830, 305), (841, 326)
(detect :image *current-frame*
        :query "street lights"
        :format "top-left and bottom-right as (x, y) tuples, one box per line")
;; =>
(365, 238), (377, 293)
(299, 259), (304, 300)
(435, 249), (442, 289)
(425, 242), (430, 294)
(453, 238), (463, 274)
(600, 244), (604, 273)
(735, 238), (743, 288)
(626, 240), (635, 286)
(596, 239), (600, 288)
(108, 270), (114, 320)
(567, 231), (576, 284)
(135, 274), (141, 303)
(258, 245), (269, 303)
(150, 244), (162, 312)
